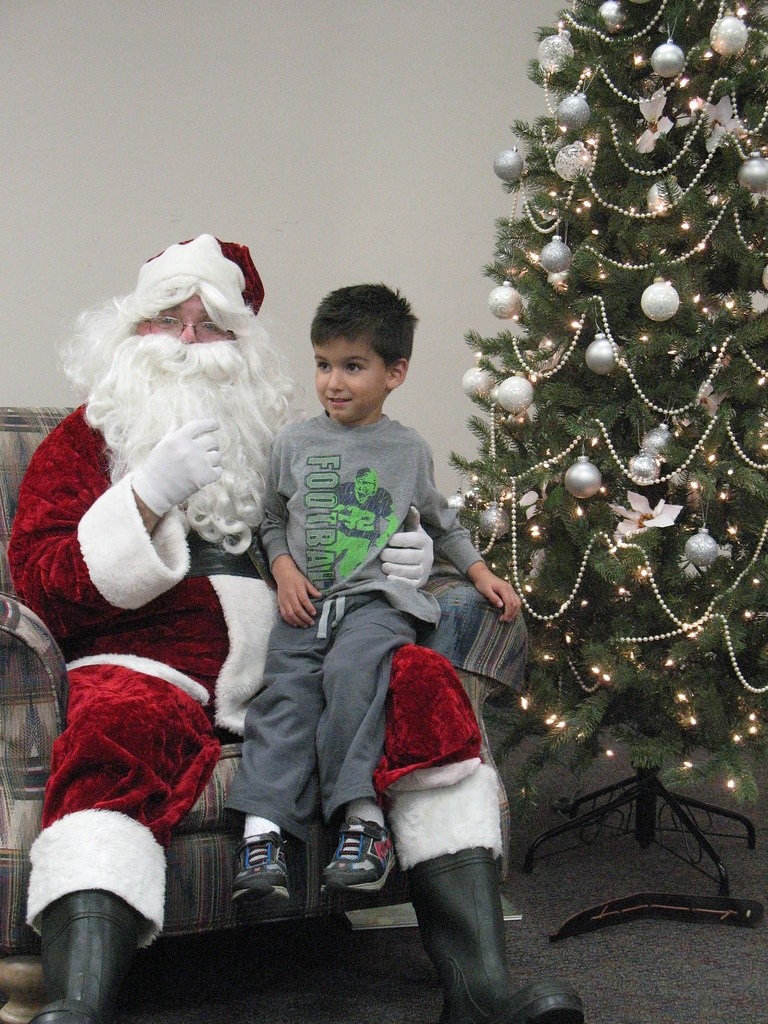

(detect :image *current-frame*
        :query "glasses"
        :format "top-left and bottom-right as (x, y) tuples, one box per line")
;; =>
(143, 316), (235, 343)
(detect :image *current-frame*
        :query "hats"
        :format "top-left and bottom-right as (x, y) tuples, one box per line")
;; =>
(137, 234), (265, 316)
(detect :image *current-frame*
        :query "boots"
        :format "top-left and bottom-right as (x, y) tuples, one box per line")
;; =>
(406, 846), (585, 1024)
(28, 890), (146, 1024)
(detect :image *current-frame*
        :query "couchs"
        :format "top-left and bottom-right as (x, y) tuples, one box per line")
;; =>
(0, 405), (530, 1024)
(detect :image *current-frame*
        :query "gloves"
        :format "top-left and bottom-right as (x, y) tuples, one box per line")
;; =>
(380, 506), (434, 589)
(129, 417), (223, 518)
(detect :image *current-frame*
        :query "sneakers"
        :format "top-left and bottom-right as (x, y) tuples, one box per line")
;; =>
(319, 815), (396, 893)
(232, 831), (290, 906)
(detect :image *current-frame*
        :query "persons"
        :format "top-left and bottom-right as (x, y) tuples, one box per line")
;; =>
(9, 233), (587, 1024)
(220, 282), (523, 908)
